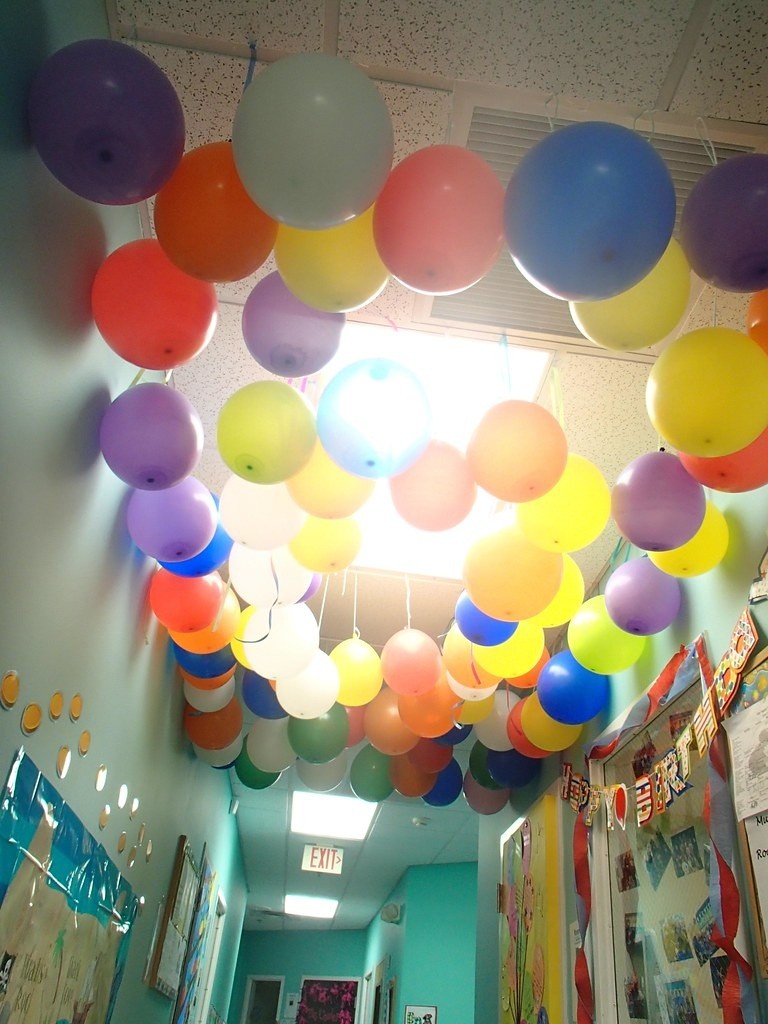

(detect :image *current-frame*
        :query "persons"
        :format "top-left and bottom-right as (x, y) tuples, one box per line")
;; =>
(614, 717), (728, 1024)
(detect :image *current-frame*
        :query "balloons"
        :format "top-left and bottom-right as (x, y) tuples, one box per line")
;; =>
(32, 40), (768, 817)
